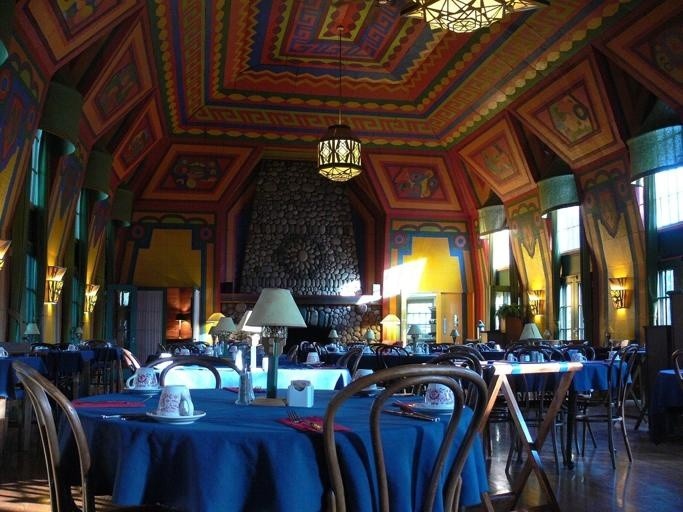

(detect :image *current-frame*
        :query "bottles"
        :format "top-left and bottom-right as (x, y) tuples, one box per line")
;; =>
(234, 364), (256, 406)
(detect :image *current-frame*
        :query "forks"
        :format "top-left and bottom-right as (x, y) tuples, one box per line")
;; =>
(285, 408), (319, 430)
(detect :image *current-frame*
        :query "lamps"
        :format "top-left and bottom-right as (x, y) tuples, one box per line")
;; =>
(74, 327), (83, 337)
(0, 239), (14, 270)
(46, 265), (66, 305)
(85, 283), (101, 313)
(175, 313), (187, 339)
(313, 26), (364, 184)
(23, 322), (39, 342)
(399, 0), (551, 36)
(206, 287), (307, 402)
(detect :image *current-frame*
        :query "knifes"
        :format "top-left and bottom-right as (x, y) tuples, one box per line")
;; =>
(385, 409), (438, 421)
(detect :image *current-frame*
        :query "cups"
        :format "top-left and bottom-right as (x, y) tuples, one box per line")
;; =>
(424, 382), (456, 408)
(125, 367), (159, 390)
(307, 352), (319, 363)
(354, 369), (377, 390)
(608, 351), (619, 359)
(180, 343), (238, 355)
(155, 384), (194, 419)
(67, 345), (78, 351)
(328, 342), (429, 355)
(575, 353), (587, 361)
(0, 346), (8, 357)
(507, 352), (518, 362)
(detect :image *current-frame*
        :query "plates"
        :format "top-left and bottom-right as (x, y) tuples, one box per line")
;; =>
(0, 356), (7, 359)
(409, 402), (455, 416)
(123, 388), (160, 396)
(355, 386), (387, 394)
(145, 409), (208, 425)
(303, 361), (325, 366)
